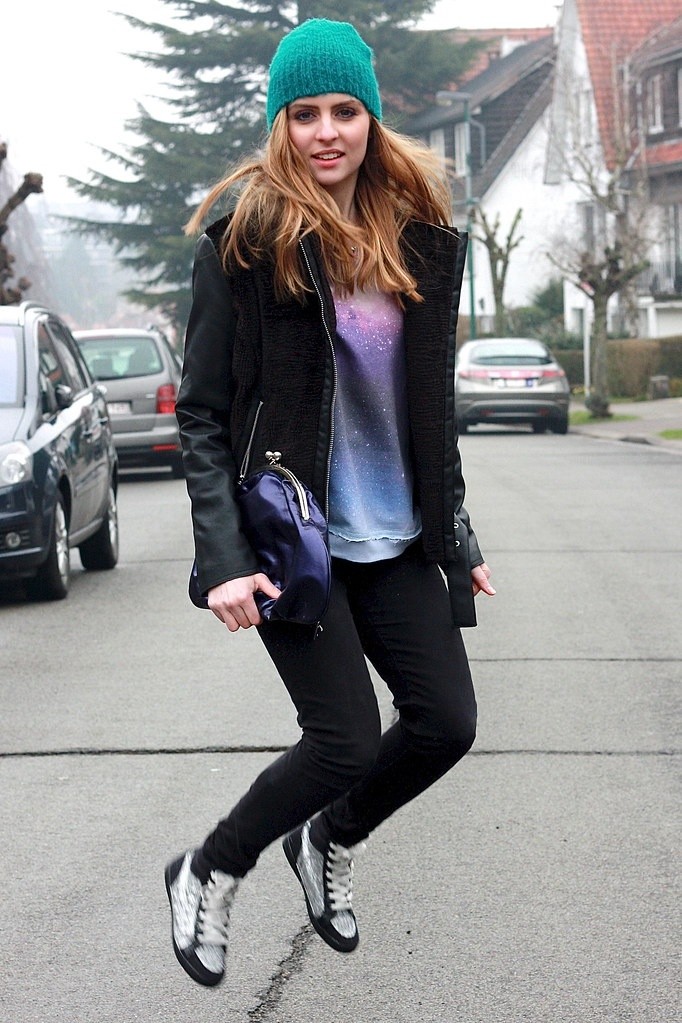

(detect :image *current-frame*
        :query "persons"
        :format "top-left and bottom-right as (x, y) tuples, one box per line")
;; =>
(160, 18), (497, 988)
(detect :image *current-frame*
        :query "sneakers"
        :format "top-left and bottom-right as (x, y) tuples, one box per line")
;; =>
(282, 814), (372, 954)
(164, 846), (242, 987)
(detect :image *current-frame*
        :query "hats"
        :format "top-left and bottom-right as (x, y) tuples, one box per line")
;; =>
(266, 18), (382, 133)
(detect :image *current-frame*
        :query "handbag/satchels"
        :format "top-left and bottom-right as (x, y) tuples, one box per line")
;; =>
(188, 450), (333, 623)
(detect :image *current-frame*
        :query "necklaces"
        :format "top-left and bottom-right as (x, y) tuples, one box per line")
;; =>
(349, 242), (358, 254)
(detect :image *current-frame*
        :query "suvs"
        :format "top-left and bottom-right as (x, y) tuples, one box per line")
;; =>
(0, 301), (118, 603)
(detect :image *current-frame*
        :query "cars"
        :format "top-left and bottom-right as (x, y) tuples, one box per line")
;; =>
(454, 339), (572, 434)
(67, 329), (188, 482)
